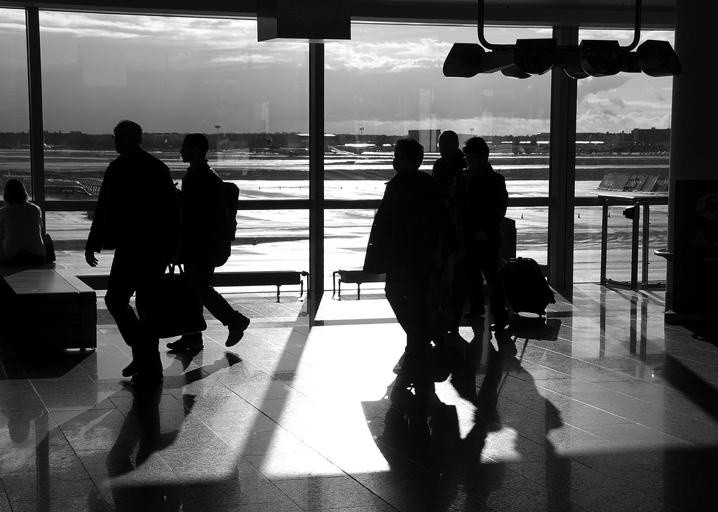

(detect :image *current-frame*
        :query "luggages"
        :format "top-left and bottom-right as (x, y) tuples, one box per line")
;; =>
(499, 252), (558, 318)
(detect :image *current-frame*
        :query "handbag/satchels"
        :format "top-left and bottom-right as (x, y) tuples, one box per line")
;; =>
(141, 271), (208, 339)
(162, 187), (192, 265)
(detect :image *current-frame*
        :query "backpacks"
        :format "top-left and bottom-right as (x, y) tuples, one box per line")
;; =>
(199, 166), (240, 266)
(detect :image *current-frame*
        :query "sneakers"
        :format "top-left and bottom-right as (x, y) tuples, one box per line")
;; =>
(225, 311), (251, 347)
(166, 332), (204, 351)
(122, 354), (163, 379)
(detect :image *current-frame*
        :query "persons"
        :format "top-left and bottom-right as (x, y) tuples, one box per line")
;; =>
(83, 118), (178, 378)
(362, 127), (518, 374)
(165, 134), (250, 352)
(0, 178), (48, 266)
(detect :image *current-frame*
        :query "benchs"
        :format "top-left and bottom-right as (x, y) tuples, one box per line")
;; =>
(1, 256), (99, 352)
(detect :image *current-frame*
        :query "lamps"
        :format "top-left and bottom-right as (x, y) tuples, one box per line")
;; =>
(442, 1), (684, 81)
